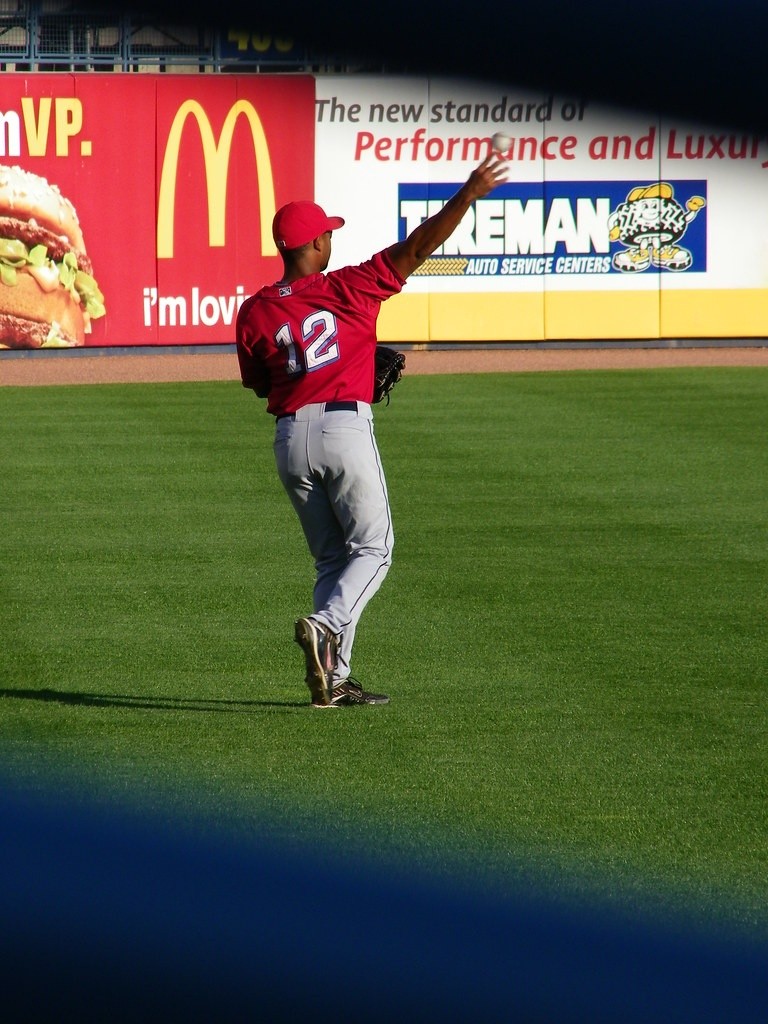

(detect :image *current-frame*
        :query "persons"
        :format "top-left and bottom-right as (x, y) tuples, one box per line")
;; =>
(236, 149), (509, 708)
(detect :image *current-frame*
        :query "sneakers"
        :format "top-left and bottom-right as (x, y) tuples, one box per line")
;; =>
(312, 675), (390, 706)
(295, 618), (337, 705)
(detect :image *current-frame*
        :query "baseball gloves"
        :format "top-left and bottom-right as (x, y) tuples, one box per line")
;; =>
(371, 345), (407, 408)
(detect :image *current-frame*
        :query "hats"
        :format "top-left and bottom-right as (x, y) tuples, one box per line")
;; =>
(272, 200), (345, 249)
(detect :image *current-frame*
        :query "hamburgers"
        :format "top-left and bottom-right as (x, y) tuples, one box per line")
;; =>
(0, 163), (108, 348)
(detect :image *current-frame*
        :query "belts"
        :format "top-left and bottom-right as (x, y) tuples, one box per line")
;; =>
(275, 401), (358, 421)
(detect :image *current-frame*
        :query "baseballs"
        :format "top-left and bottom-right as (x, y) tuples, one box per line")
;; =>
(493, 132), (511, 152)
